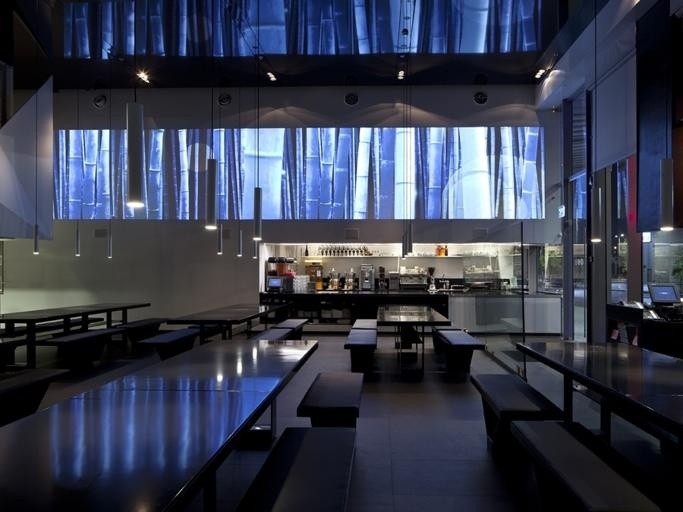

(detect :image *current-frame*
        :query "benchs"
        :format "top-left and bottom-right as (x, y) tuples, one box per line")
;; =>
(509, 419), (682, 512)
(0, 303), (310, 370)
(233, 424), (355, 512)
(344, 319), (378, 372)
(296, 371), (363, 426)
(470, 373), (565, 460)
(431, 326), (486, 378)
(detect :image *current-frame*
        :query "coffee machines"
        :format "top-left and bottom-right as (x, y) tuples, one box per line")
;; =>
(427, 265), (436, 290)
(359, 264), (375, 290)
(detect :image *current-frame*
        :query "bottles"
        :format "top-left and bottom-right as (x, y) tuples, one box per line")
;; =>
(303, 245), (308, 256)
(317, 245), (366, 256)
(314, 268), (355, 291)
(434, 245), (448, 256)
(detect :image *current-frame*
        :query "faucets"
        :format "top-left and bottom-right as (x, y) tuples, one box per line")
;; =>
(439, 280), (449, 289)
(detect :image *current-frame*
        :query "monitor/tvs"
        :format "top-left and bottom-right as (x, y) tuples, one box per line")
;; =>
(647, 282), (680, 303)
(266, 275), (284, 288)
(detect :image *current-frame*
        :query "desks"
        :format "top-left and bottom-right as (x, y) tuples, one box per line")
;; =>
(0, 338), (321, 512)
(1, 367), (72, 427)
(376, 305), (451, 382)
(515, 339), (682, 478)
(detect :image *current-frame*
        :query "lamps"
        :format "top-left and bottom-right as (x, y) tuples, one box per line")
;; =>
(253, 6), (263, 258)
(33, 37), (40, 254)
(74, 39), (81, 258)
(398, 1), (415, 261)
(590, 1), (602, 245)
(205, 21), (217, 231)
(107, 37), (113, 260)
(236, 86), (243, 258)
(126, 2), (146, 209)
(659, 1), (674, 234)
(216, 100), (224, 256)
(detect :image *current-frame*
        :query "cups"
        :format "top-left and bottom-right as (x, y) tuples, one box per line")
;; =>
(371, 249), (383, 256)
(400, 266), (423, 273)
(510, 276), (517, 286)
(293, 274), (311, 289)
